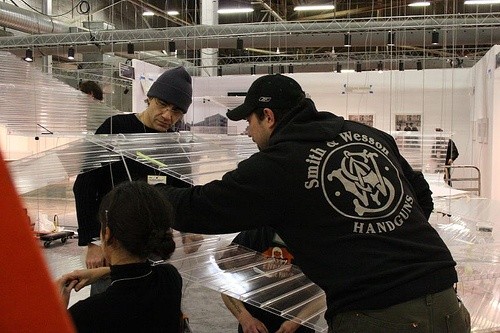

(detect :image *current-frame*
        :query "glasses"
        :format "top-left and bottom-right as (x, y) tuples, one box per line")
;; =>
(154, 97), (184, 119)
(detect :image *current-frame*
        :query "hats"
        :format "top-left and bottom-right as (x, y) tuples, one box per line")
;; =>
(226, 73), (306, 121)
(147, 66), (192, 114)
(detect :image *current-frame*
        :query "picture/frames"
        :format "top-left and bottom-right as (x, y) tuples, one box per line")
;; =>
(393, 113), (423, 152)
(347, 113), (375, 128)
(470, 121), (477, 141)
(477, 117), (489, 144)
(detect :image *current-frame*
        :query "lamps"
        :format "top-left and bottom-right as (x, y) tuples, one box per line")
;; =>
(387, 30), (396, 47)
(278, 64), (284, 74)
(68, 47), (75, 60)
(337, 62), (342, 73)
(357, 61), (362, 72)
(216, 66), (223, 77)
(237, 38), (244, 50)
(289, 64), (294, 73)
(344, 32), (352, 47)
(24, 47), (34, 62)
(127, 42), (135, 55)
(251, 65), (256, 75)
(417, 60), (423, 70)
(377, 61), (383, 71)
(124, 87), (129, 95)
(168, 40), (175, 52)
(431, 31), (440, 45)
(268, 65), (274, 74)
(399, 60), (404, 71)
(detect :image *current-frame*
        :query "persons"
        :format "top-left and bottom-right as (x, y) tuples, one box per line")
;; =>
(52, 179), (183, 333)
(153, 73), (472, 333)
(403, 122), (422, 147)
(76, 79), (115, 154)
(73, 65), (204, 297)
(217, 226), (327, 333)
(431, 128), (460, 188)
